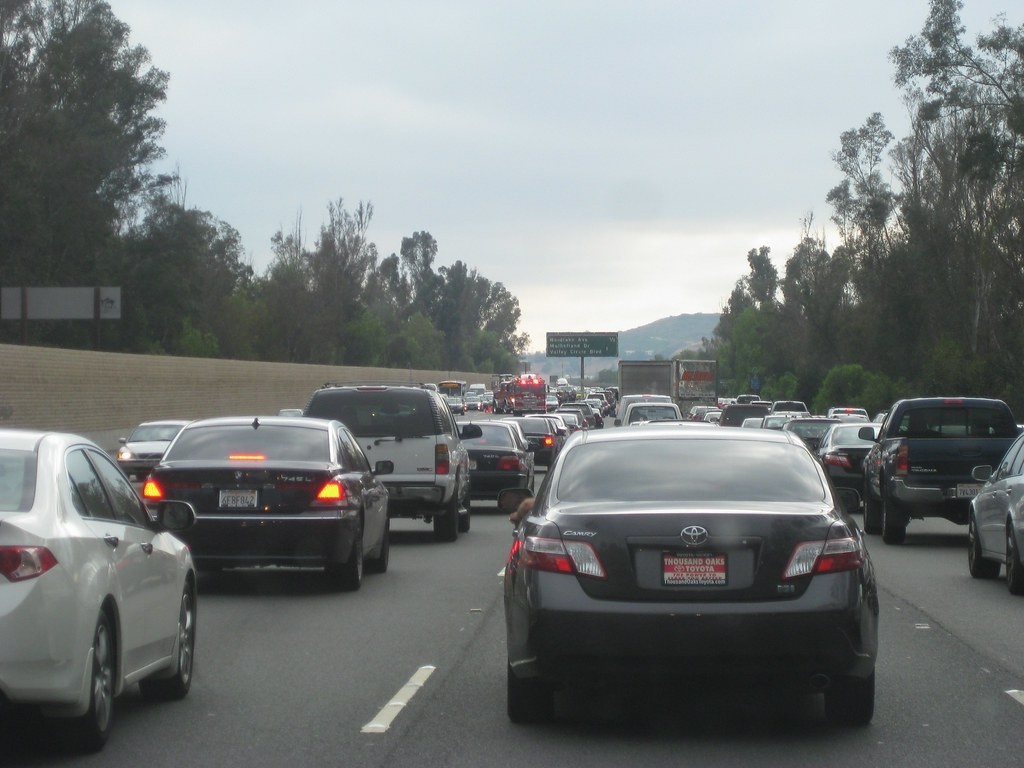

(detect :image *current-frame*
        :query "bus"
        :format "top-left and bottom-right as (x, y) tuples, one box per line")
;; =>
(436, 380), (468, 415)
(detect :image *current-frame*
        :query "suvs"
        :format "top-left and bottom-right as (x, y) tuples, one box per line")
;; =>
(299, 383), (483, 542)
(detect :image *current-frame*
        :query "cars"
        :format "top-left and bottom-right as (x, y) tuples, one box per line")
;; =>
(0, 428), (198, 753)
(459, 377), (907, 515)
(132, 415), (395, 592)
(116, 420), (191, 481)
(496, 422), (880, 729)
(968, 429), (1024, 596)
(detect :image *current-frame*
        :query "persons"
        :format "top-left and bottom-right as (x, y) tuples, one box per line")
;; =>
(500, 492), (520, 510)
(510, 497), (535, 523)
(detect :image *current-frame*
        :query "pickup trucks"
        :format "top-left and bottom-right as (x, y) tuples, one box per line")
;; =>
(858, 395), (1020, 547)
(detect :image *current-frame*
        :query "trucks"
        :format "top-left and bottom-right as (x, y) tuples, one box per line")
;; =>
(676, 356), (720, 417)
(612, 359), (677, 414)
